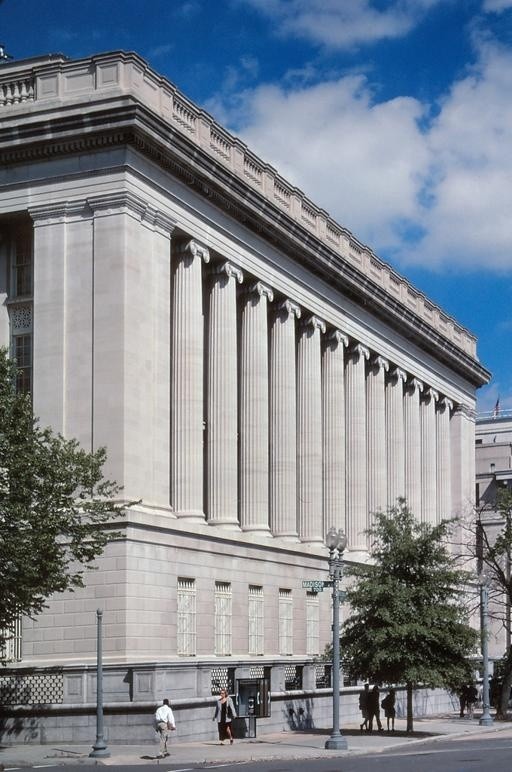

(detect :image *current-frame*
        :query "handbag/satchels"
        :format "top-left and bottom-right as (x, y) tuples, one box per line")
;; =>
(223, 706), (233, 719)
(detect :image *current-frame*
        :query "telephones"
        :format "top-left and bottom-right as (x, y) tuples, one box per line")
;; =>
(248, 696), (257, 709)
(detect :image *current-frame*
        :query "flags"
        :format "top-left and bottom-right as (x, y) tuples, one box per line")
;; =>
(491, 393), (504, 418)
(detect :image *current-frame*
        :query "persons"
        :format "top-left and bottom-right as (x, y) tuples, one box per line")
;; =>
(356, 683), (396, 733)
(155, 698), (176, 758)
(212, 689), (238, 746)
(456, 675), (510, 718)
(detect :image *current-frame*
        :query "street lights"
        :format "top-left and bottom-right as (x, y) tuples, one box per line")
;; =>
(478, 568), (494, 726)
(325, 527), (348, 749)
